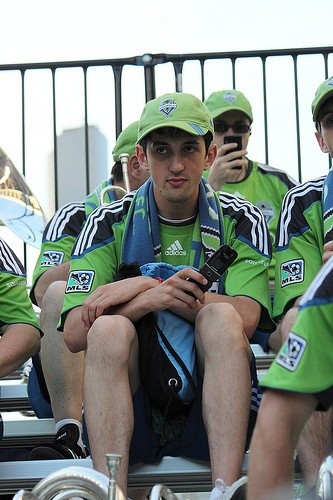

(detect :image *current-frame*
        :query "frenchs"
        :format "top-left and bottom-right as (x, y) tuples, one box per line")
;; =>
(9, 452), (129, 500)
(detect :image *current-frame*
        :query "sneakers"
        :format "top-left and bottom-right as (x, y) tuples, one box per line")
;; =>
(29, 422), (91, 461)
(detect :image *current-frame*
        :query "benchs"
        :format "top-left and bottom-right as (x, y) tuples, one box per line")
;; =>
(0, 343), (306, 500)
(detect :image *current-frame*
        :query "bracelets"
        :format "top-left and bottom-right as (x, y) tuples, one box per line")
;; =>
(155, 277), (163, 287)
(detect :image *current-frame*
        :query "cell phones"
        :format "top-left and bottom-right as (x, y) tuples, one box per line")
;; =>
(180, 244), (238, 300)
(224, 135), (242, 171)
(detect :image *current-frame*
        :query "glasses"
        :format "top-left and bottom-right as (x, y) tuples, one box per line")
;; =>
(212, 121), (251, 134)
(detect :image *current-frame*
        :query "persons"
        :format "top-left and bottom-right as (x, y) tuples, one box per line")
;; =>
(53, 92), (278, 500)
(273, 72), (333, 491)
(0, 237), (45, 451)
(245, 247), (332, 500)
(29, 120), (151, 465)
(199, 90), (306, 353)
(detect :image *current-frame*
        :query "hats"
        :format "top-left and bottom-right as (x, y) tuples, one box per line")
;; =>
(311, 77), (333, 121)
(134, 93), (216, 150)
(201, 90), (253, 125)
(112, 121), (140, 161)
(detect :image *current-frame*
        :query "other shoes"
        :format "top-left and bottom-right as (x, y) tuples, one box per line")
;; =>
(212, 475), (247, 500)
